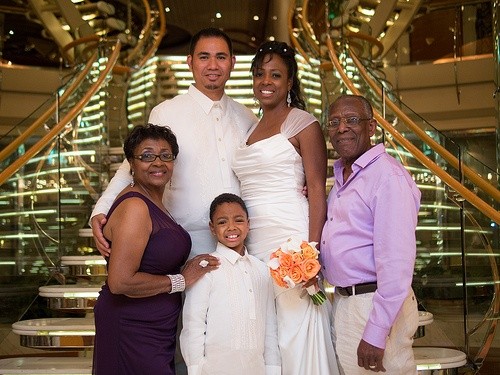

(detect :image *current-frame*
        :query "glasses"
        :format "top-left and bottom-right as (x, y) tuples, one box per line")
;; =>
(133, 153), (175, 162)
(328, 117), (370, 127)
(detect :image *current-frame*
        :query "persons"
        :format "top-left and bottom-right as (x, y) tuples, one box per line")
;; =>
(302, 95), (422, 375)
(230, 41), (340, 375)
(87, 27), (308, 301)
(91, 123), (221, 375)
(179, 193), (283, 375)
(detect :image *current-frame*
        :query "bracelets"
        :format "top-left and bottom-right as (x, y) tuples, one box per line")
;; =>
(167, 273), (185, 294)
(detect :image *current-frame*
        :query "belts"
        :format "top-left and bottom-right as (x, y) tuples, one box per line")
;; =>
(337, 283), (377, 296)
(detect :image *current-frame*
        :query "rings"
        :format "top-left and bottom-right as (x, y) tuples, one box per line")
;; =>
(199, 260), (209, 267)
(368, 363), (377, 369)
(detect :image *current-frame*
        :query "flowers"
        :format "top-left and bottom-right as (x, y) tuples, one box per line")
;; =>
(268, 238), (328, 306)
